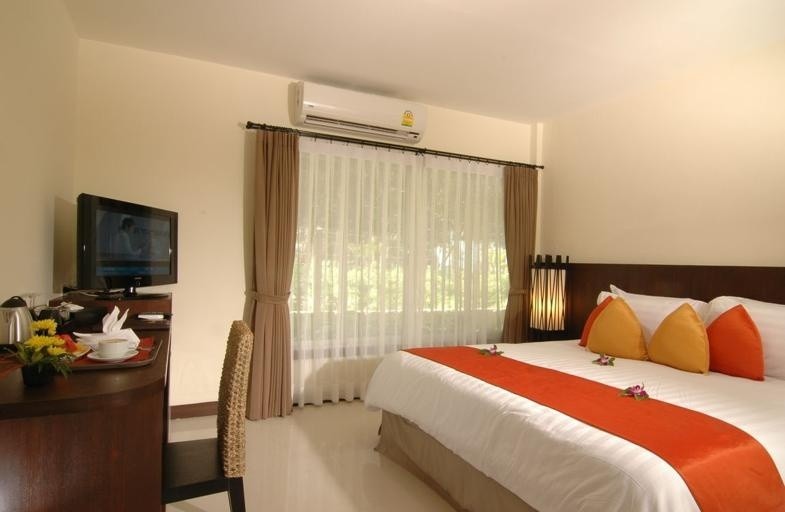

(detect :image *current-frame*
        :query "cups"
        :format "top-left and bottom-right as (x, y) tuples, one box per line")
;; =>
(92, 339), (130, 359)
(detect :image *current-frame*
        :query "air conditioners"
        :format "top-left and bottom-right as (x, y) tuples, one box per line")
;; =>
(288, 80), (425, 147)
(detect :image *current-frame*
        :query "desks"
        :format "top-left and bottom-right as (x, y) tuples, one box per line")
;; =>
(0, 291), (172, 512)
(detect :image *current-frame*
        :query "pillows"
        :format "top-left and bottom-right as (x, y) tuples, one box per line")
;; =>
(707, 296), (785, 380)
(584, 296), (650, 363)
(578, 295), (616, 348)
(646, 303), (711, 375)
(596, 289), (708, 350)
(705, 303), (764, 380)
(610, 283), (709, 320)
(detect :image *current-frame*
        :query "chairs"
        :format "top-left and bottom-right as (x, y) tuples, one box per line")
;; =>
(162, 318), (255, 511)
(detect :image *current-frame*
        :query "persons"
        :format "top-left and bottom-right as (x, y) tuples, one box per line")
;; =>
(110, 216), (149, 269)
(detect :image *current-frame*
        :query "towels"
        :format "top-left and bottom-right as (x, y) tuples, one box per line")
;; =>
(71, 304), (140, 354)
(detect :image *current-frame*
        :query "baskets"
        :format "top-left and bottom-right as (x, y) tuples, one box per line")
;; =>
(137, 311), (165, 327)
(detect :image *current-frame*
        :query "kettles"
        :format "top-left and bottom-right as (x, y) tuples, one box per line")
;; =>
(1, 296), (37, 355)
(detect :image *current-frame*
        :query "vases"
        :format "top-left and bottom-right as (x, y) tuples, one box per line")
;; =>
(21, 364), (54, 393)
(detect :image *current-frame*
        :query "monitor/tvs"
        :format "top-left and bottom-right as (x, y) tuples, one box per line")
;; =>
(76, 193), (178, 300)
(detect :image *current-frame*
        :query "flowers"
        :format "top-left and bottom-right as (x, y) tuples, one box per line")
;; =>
(479, 343), (506, 358)
(619, 381), (651, 402)
(592, 352), (614, 367)
(0, 319), (77, 384)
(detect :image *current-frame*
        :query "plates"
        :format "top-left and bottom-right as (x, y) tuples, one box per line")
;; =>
(88, 349), (140, 364)
(52, 343), (92, 363)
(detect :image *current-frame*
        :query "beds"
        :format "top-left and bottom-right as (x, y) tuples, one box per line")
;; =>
(361, 284), (784, 510)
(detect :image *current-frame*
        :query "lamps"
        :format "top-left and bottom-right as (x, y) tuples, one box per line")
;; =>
(525, 252), (571, 343)
